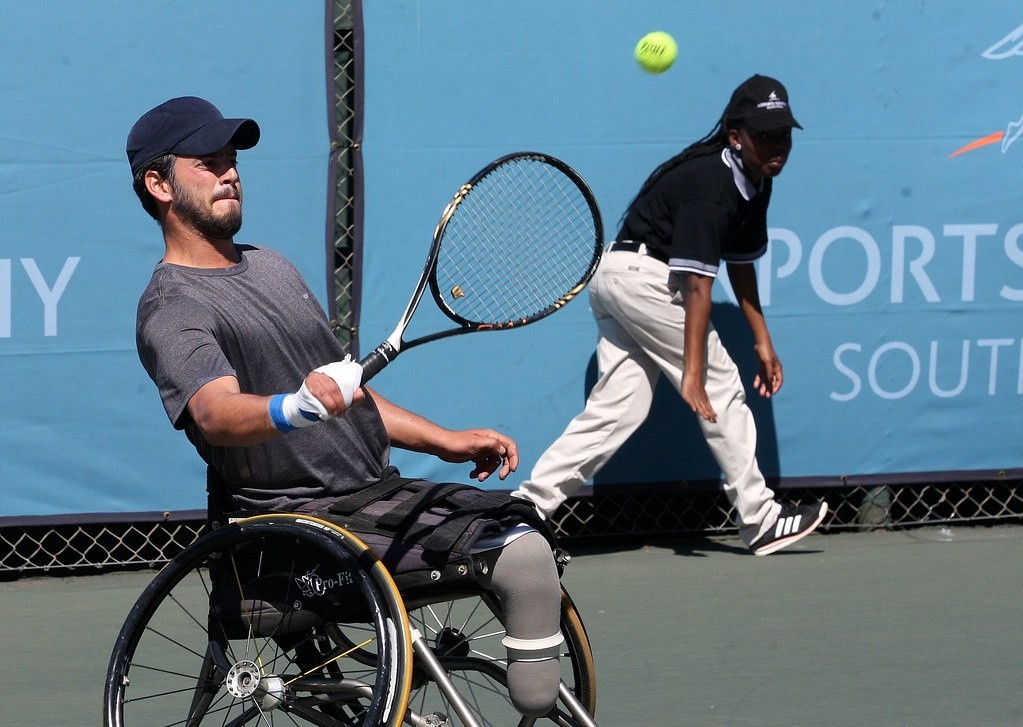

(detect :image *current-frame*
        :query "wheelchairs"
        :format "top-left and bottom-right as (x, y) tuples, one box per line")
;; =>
(100, 452), (599, 727)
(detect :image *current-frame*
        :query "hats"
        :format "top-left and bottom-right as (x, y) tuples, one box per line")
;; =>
(128, 95), (262, 168)
(729, 72), (807, 136)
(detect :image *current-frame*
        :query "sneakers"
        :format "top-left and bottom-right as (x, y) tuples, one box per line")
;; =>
(751, 501), (828, 554)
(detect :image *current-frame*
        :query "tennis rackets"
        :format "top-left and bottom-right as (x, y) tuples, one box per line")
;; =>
(300, 148), (605, 422)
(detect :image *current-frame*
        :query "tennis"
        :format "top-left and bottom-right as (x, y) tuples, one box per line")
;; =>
(633, 29), (681, 73)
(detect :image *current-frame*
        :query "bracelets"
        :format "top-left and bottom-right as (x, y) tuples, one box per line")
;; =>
(268, 392), (298, 434)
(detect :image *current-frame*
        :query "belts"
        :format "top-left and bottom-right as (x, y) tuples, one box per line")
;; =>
(606, 242), (670, 266)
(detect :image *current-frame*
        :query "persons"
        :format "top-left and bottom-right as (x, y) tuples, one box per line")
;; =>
(125, 96), (564, 717)
(510, 75), (827, 557)
